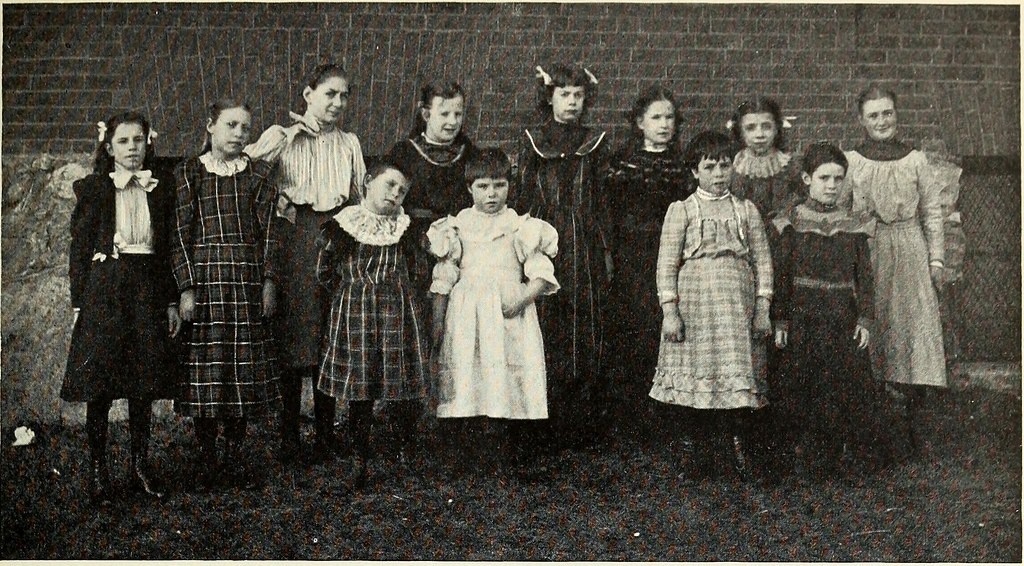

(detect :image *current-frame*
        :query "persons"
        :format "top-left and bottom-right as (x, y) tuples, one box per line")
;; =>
(842, 87), (948, 453)
(244, 63), (367, 465)
(315, 155), (430, 494)
(609, 87), (696, 444)
(169, 97), (277, 492)
(513, 65), (609, 454)
(390, 80), (480, 228)
(426, 151), (561, 483)
(60, 112), (183, 508)
(729, 96), (802, 215)
(647, 130), (774, 482)
(768, 143), (875, 487)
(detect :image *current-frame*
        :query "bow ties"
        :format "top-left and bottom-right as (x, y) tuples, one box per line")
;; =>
(109, 170), (159, 192)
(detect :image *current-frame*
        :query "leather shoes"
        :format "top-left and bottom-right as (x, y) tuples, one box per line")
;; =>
(131, 461), (167, 497)
(91, 459), (113, 507)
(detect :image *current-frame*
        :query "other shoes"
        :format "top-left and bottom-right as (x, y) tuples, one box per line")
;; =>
(734, 436), (755, 482)
(678, 441), (694, 480)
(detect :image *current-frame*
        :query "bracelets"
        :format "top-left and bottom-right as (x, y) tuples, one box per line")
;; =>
(929, 260), (943, 269)
